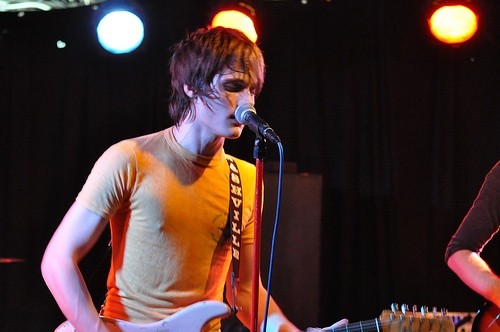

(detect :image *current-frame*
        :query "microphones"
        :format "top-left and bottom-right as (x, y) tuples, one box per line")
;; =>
(234, 103), (282, 144)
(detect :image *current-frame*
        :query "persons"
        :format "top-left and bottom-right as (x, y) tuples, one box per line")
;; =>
(446, 159), (500, 308)
(40, 26), (348, 332)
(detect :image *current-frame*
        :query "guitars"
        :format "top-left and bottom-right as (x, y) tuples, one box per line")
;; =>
(55, 300), (456, 332)
(471, 301), (500, 332)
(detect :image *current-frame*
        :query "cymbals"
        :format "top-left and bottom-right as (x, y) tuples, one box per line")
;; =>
(0, 258), (30, 263)
